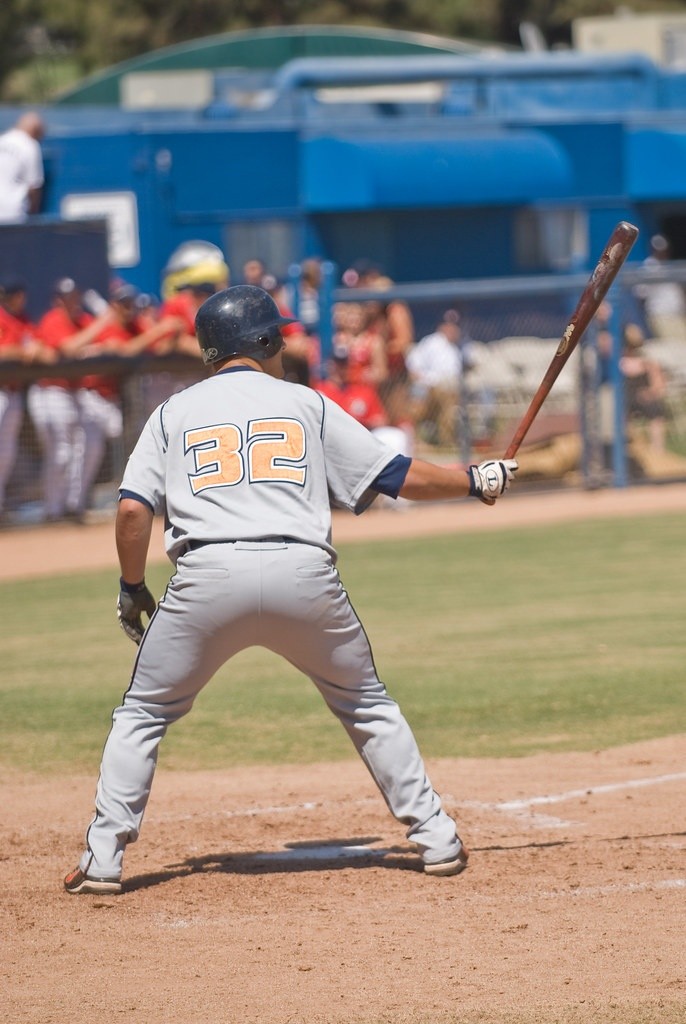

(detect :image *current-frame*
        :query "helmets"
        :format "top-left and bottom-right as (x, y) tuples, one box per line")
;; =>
(194, 285), (300, 365)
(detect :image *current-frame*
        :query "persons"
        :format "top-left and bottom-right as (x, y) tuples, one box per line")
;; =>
(63, 283), (519, 896)
(0, 111), (685, 522)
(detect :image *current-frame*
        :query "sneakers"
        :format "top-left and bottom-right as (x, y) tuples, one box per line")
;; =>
(424, 841), (469, 875)
(64, 866), (122, 893)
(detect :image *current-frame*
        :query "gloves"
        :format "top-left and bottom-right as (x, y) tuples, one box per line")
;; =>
(466, 459), (518, 500)
(115, 577), (156, 645)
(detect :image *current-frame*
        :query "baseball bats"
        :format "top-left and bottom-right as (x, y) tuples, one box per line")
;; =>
(478, 221), (641, 506)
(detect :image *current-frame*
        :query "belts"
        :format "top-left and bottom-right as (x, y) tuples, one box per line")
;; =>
(184, 537), (296, 554)
